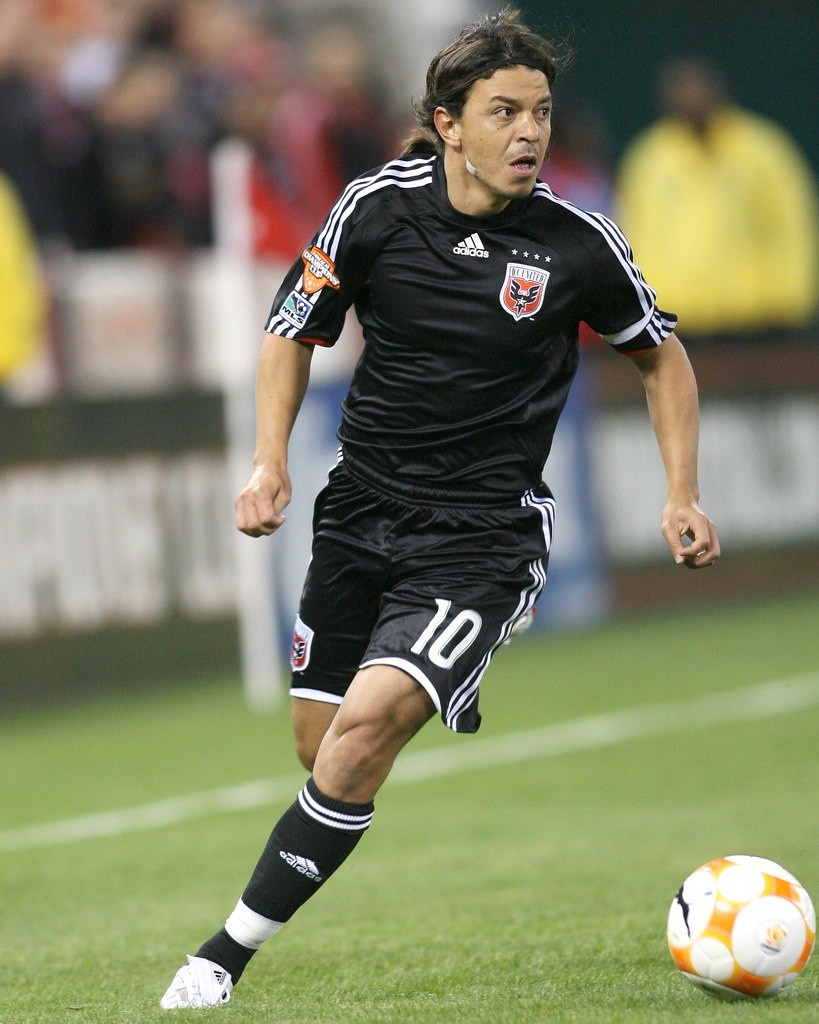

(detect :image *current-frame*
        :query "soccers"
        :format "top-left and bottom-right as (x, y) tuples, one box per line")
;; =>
(668, 853), (816, 998)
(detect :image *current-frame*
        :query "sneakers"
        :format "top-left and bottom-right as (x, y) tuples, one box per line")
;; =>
(158, 950), (234, 1010)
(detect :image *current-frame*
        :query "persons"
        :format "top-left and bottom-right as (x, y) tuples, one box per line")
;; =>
(0, 0), (819, 410)
(161, 17), (721, 1010)
